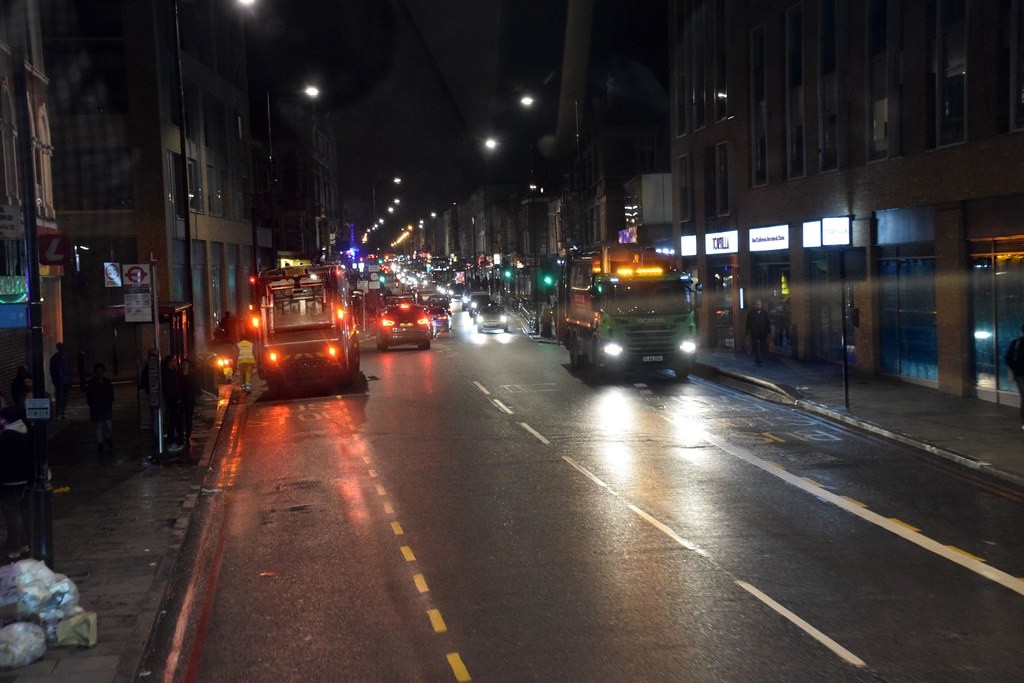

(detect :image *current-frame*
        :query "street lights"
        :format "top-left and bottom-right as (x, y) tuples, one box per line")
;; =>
(520, 93), (586, 247)
(485, 134), (538, 333)
(266, 86), (320, 264)
(173, 0), (259, 379)
(372, 177), (401, 261)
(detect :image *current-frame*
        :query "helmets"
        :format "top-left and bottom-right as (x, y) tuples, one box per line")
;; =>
(215, 328), (225, 336)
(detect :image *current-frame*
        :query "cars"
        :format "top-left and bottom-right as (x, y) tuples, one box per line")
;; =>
(462, 289), (508, 333)
(378, 261), (452, 333)
(375, 303), (431, 350)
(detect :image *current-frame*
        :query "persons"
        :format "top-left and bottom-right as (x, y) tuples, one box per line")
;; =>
(0, 341), (76, 558)
(745, 299), (772, 366)
(1005, 326), (1024, 430)
(143, 311), (255, 453)
(86, 363), (116, 455)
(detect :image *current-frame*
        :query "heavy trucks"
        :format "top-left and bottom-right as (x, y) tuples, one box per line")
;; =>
(556, 245), (695, 380)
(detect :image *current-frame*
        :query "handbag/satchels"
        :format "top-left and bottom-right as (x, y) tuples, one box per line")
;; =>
(742, 334), (752, 357)
(50, 370), (69, 385)
(1008, 369), (1015, 384)
(768, 335), (775, 352)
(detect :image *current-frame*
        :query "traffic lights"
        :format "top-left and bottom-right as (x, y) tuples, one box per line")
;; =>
(545, 274), (554, 294)
(505, 269), (512, 284)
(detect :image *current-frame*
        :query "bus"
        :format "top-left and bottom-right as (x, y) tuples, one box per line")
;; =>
(249, 263), (359, 387)
(430, 262), (467, 296)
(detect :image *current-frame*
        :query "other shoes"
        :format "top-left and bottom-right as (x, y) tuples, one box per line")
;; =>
(105, 437), (114, 448)
(20, 550), (31, 556)
(56, 414), (67, 421)
(186, 436), (191, 448)
(246, 390), (251, 394)
(97, 443), (104, 454)
(167, 443), (183, 451)
(243, 386), (246, 393)
(755, 360), (763, 367)
(2, 553), (22, 564)
